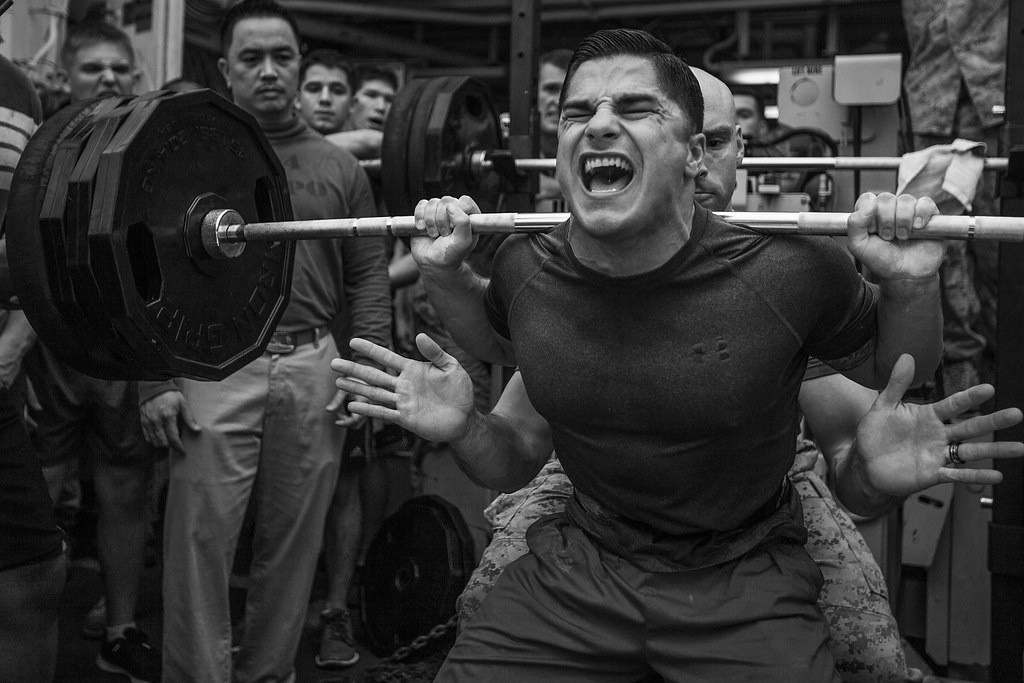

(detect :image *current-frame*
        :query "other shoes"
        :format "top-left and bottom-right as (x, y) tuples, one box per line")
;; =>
(81, 597), (108, 638)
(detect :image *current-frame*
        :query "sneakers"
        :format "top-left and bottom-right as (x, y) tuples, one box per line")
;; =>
(225, 601), (251, 651)
(314, 608), (360, 667)
(95, 627), (163, 683)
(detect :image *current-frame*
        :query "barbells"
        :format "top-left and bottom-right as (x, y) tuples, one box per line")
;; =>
(380, 74), (1024, 256)
(4, 87), (1024, 382)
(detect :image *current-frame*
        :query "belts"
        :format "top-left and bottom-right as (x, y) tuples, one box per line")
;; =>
(265, 323), (330, 355)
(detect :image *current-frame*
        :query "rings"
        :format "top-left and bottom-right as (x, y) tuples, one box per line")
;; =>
(949, 441), (967, 466)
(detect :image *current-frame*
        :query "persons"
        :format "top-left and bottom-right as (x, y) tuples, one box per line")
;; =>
(330, 0), (1024, 682)
(291, 47), (397, 668)
(137, 0), (392, 683)
(0, 0), (208, 683)
